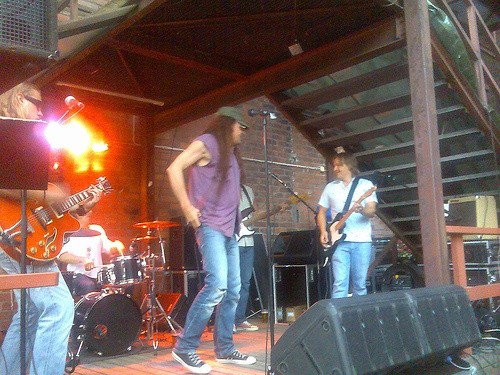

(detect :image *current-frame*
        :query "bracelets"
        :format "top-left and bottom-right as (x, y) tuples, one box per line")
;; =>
(77, 204), (88, 216)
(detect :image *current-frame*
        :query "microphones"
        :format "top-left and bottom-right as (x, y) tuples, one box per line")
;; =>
(129, 234), (138, 252)
(248, 109), (271, 116)
(65, 95), (84, 107)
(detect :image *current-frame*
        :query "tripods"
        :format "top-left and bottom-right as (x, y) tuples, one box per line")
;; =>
(133, 228), (183, 354)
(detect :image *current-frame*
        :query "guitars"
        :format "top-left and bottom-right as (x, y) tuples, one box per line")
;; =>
(319, 184), (378, 252)
(0, 175), (115, 267)
(233, 193), (311, 243)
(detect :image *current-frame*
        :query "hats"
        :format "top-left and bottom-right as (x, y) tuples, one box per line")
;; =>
(217, 106), (247, 126)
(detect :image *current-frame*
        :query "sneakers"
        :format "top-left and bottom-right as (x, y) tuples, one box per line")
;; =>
(233, 321), (258, 333)
(171, 349), (211, 374)
(215, 348), (256, 365)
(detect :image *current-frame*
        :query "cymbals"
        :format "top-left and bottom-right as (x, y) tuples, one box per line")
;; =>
(131, 221), (182, 228)
(130, 236), (168, 243)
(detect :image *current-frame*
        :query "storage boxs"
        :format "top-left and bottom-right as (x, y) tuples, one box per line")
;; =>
(170, 269), (206, 299)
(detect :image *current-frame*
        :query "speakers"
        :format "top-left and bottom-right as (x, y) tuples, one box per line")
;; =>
(168, 226), (202, 269)
(271, 285), (481, 375)
(272, 229), (325, 266)
(446, 195), (499, 322)
(142, 293), (191, 330)
(0, 0), (59, 74)
(165, 269), (216, 325)
(0, 117), (50, 190)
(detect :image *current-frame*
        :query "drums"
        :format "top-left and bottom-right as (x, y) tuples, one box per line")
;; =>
(96, 263), (129, 289)
(111, 255), (145, 285)
(71, 291), (143, 357)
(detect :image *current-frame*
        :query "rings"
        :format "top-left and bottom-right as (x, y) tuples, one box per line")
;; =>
(97, 195), (99, 199)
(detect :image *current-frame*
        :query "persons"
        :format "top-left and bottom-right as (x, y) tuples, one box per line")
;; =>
(0, 79), (103, 375)
(167, 107), (256, 375)
(233, 168), (258, 333)
(317, 152), (378, 298)
(57, 209), (119, 295)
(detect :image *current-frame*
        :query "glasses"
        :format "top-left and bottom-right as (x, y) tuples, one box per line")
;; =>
(22, 94), (44, 111)
